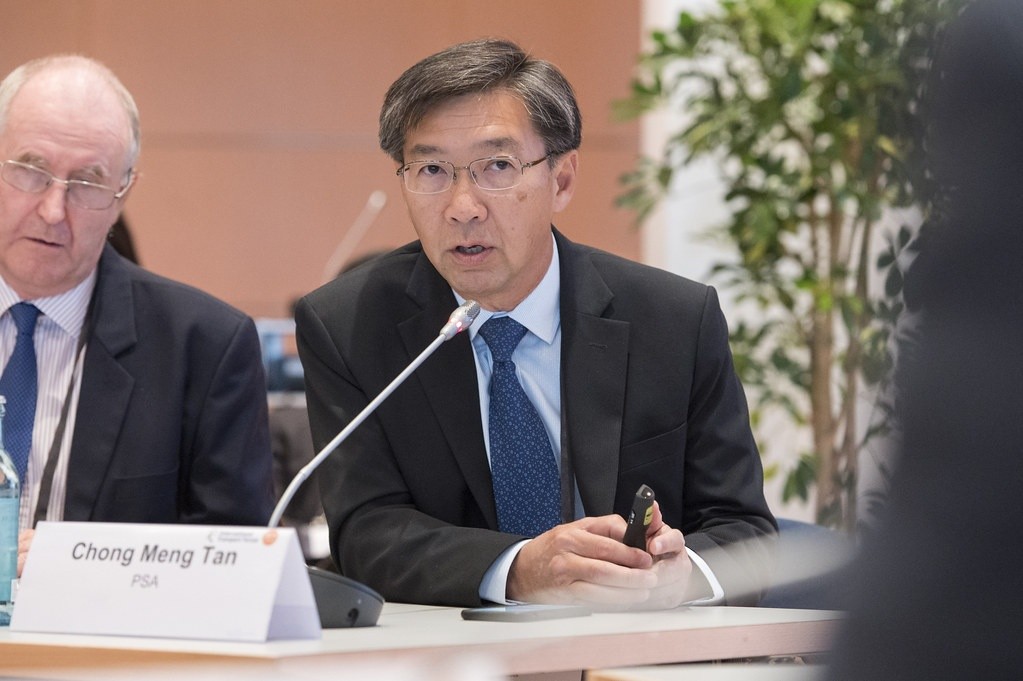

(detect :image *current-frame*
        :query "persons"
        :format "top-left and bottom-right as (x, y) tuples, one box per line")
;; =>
(821, 0), (1023, 681)
(295, 38), (779, 611)
(0, 55), (290, 577)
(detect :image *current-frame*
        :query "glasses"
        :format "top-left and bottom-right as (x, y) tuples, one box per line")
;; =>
(0, 160), (135, 210)
(397, 149), (557, 196)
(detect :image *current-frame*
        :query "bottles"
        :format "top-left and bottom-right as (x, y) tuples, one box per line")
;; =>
(0, 395), (22, 626)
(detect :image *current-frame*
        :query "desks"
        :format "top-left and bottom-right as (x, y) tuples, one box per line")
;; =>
(0, 602), (844, 681)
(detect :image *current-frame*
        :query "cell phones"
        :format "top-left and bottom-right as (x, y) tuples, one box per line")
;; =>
(459, 603), (589, 624)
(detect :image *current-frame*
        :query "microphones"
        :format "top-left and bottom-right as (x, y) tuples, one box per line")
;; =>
(265, 299), (481, 528)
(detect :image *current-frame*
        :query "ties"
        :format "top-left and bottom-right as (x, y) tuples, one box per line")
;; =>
(0, 301), (45, 498)
(480, 316), (562, 538)
